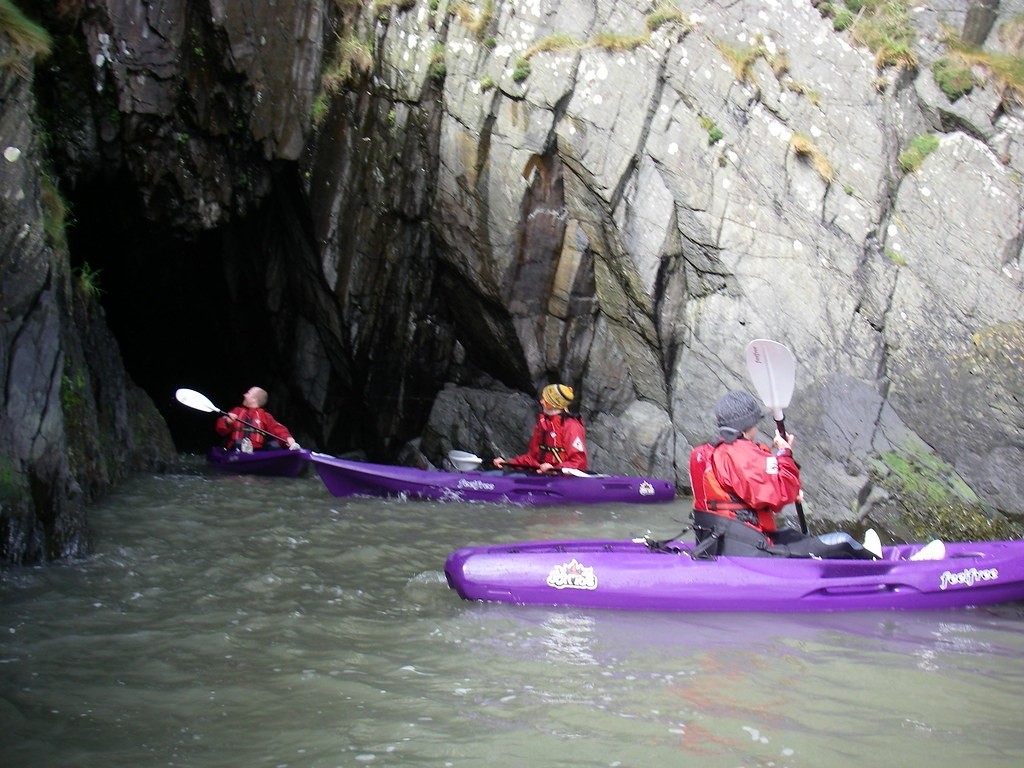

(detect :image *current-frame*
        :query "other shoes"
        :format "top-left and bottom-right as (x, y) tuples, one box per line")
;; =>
(241, 437), (253, 453)
(416, 450), (437, 472)
(442, 458), (458, 472)
(289, 443), (300, 451)
(863, 527), (882, 558)
(909, 539), (945, 562)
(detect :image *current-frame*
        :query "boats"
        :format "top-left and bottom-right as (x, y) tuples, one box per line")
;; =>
(302, 451), (677, 509)
(445, 540), (1023, 613)
(207, 444), (316, 479)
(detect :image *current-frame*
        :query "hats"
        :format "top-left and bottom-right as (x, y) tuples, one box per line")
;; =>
(542, 384), (575, 410)
(715, 390), (761, 432)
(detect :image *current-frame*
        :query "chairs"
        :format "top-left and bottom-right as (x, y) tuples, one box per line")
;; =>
(690, 509), (783, 558)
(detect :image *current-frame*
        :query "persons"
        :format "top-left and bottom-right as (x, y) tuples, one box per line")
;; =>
(493, 383), (589, 476)
(691, 389), (947, 562)
(216, 387), (296, 454)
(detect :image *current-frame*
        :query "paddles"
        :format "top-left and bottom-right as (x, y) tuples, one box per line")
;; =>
(174, 387), (287, 443)
(447, 448), (611, 480)
(744, 338), (810, 536)
